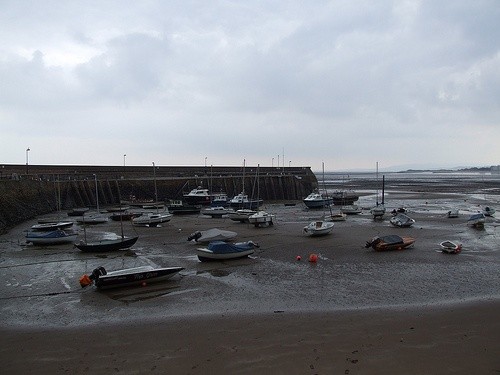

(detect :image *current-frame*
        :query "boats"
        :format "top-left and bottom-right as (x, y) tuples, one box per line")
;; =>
(440, 240), (458, 253)
(390, 210), (415, 228)
(303, 220), (334, 237)
(201, 206), (233, 219)
(91, 264), (185, 291)
(341, 204), (362, 214)
(466, 218), (486, 230)
(370, 235), (415, 252)
(302, 187), (333, 209)
(196, 239), (260, 262)
(227, 209), (258, 221)
(447, 209), (458, 218)
(187, 227), (238, 243)
(332, 174), (359, 205)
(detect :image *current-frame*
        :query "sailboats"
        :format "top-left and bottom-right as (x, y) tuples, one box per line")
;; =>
(24, 151), (211, 253)
(477, 175), (495, 216)
(228, 158), (264, 210)
(321, 162), (347, 222)
(210, 173), (231, 207)
(248, 165), (275, 229)
(369, 161), (387, 216)
